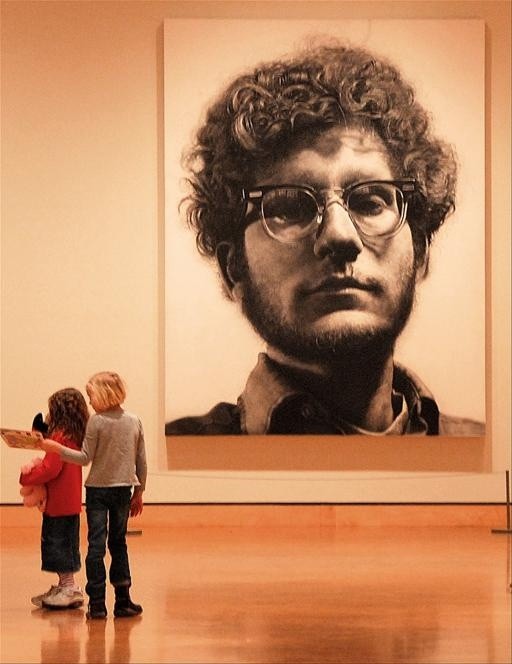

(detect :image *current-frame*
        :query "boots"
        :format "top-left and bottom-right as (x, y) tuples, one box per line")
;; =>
(89, 604), (107, 619)
(114, 585), (143, 617)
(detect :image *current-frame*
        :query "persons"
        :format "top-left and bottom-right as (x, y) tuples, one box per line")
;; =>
(166, 46), (485, 437)
(19, 370), (148, 619)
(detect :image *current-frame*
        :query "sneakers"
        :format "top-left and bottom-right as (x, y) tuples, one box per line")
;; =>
(31, 585), (85, 609)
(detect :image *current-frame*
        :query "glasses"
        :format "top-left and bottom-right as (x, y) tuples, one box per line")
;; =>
(242, 178), (416, 243)
